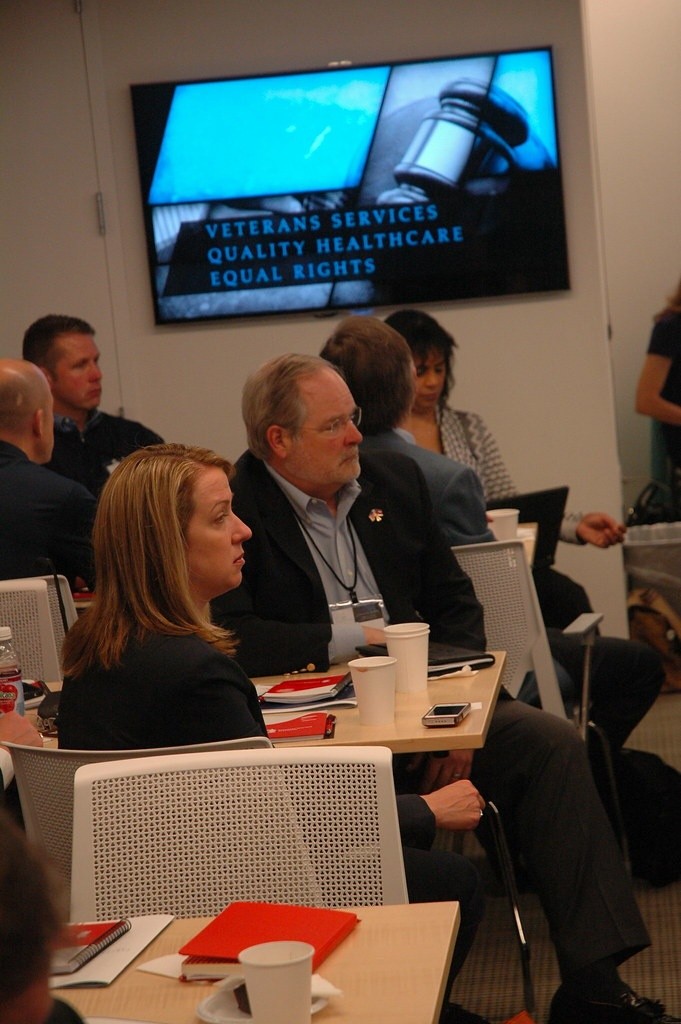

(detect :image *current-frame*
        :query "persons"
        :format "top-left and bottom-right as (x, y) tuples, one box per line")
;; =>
(0, 277), (681, 1024)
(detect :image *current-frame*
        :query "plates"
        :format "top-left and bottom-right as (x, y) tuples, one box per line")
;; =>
(196, 980), (328, 1024)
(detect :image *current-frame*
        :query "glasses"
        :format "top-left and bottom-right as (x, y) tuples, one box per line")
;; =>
(292, 407), (361, 436)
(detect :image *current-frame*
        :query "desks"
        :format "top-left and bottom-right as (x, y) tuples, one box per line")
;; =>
(47, 902), (461, 1024)
(25, 649), (507, 754)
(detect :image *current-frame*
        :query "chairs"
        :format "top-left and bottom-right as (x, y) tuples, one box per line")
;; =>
(0, 542), (604, 923)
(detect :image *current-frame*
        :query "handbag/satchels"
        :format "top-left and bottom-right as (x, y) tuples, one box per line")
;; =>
(625, 483), (681, 526)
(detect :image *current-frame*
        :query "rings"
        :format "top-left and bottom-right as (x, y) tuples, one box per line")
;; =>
(480, 810), (483, 817)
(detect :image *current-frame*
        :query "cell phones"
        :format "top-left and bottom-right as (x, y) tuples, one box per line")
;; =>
(422, 702), (471, 727)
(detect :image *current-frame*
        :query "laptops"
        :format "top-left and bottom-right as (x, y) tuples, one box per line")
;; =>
(485, 486), (569, 570)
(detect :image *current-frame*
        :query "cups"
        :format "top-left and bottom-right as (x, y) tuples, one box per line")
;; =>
(384, 622), (431, 694)
(237, 940), (314, 1024)
(485, 508), (520, 544)
(347, 655), (398, 726)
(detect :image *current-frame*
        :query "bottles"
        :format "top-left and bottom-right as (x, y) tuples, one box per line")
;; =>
(0, 626), (26, 722)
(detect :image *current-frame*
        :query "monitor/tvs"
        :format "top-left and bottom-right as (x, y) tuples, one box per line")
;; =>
(131, 47), (570, 328)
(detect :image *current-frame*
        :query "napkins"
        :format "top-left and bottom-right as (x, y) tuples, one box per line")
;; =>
(214, 973), (341, 998)
(427, 665), (479, 680)
(136, 953), (208, 985)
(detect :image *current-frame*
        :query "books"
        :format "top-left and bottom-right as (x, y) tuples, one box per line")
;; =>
(48, 915), (174, 990)
(263, 708), (329, 744)
(50, 919), (133, 975)
(178, 903), (359, 982)
(355, 644), (496, 678)
(261, 668), (357, 704)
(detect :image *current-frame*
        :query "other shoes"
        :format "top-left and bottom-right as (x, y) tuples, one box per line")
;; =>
(549, 973), (680, 1024)
